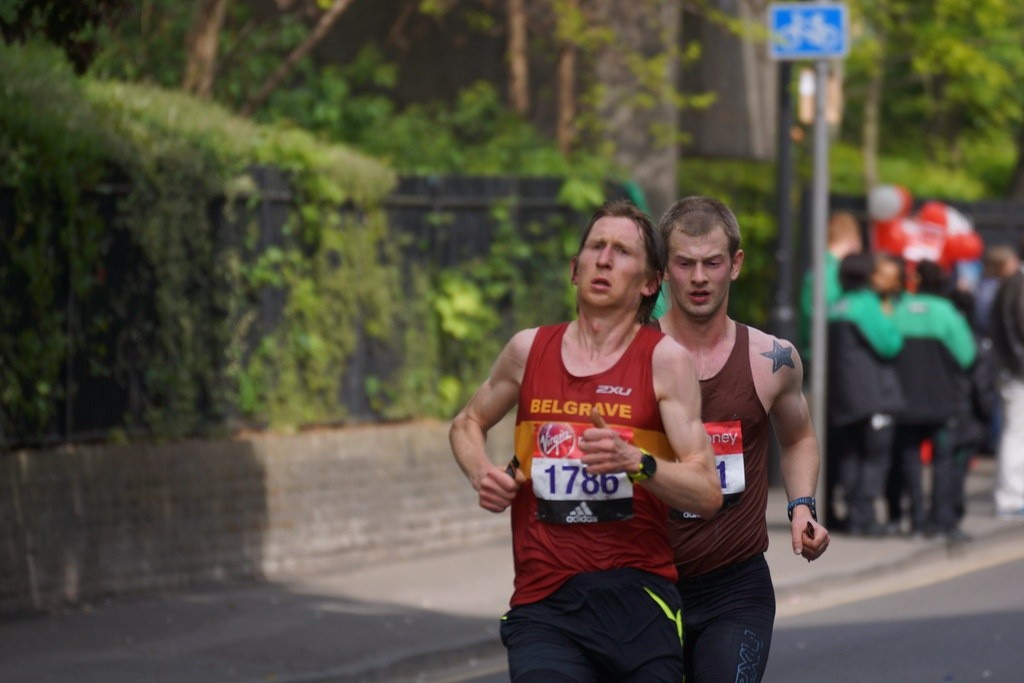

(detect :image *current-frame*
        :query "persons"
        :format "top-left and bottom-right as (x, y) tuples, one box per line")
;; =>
(646, 196), (831, 683)
(450, 203), (722, 683)
(800, 185), (1024, 538)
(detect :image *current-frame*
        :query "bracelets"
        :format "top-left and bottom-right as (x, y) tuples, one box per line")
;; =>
(788, 497), (817, 522)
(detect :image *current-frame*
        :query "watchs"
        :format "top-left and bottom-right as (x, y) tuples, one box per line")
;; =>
(626, 448), (655, 483)
